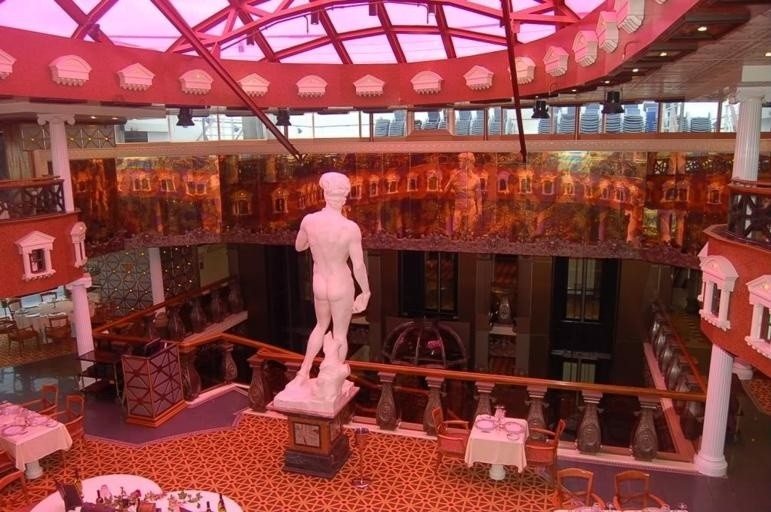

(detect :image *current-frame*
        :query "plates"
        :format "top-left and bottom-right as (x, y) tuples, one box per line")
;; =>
(503, 422), (524, 441)
(47, 311), (66, 318)
(2, 426), (23, 436)
(24, 312), (41, 318)
(474, 419), (498, 431)
(16, 309), (29, 314)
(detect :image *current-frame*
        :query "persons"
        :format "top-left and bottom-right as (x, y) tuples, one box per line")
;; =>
(284, 170), (372, 400)
(443, 151), (483, 238)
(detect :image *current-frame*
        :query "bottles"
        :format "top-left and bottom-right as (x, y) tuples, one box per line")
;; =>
(96, 491), (103, 503)
(217, 493), (226, 512)
(205, 501), (213, 512)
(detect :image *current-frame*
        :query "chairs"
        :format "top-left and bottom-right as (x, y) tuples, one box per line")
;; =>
(0, 385), (84, 506)
(0, 291), (108, 356)
(556, 468), (670, 512)
(525, 419), (568, 488)
(432, 407), (472, 476)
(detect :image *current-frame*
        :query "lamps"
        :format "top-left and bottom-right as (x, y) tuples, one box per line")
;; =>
(531, 101), (550, 118)
(275, 110), (291, 125)
(176, 107), (195, 126)
(601, 91), (625, 113)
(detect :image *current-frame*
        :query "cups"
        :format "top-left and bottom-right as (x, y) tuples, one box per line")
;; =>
(592, 503), (601, 512)
(101, 485), (109, 497)
(661, 504), (669, 512)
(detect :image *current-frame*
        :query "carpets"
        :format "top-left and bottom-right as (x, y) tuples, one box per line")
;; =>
(740, 370), (771, 415)
(0, 413), (590, 512)
(0, 315), (78, 366)
